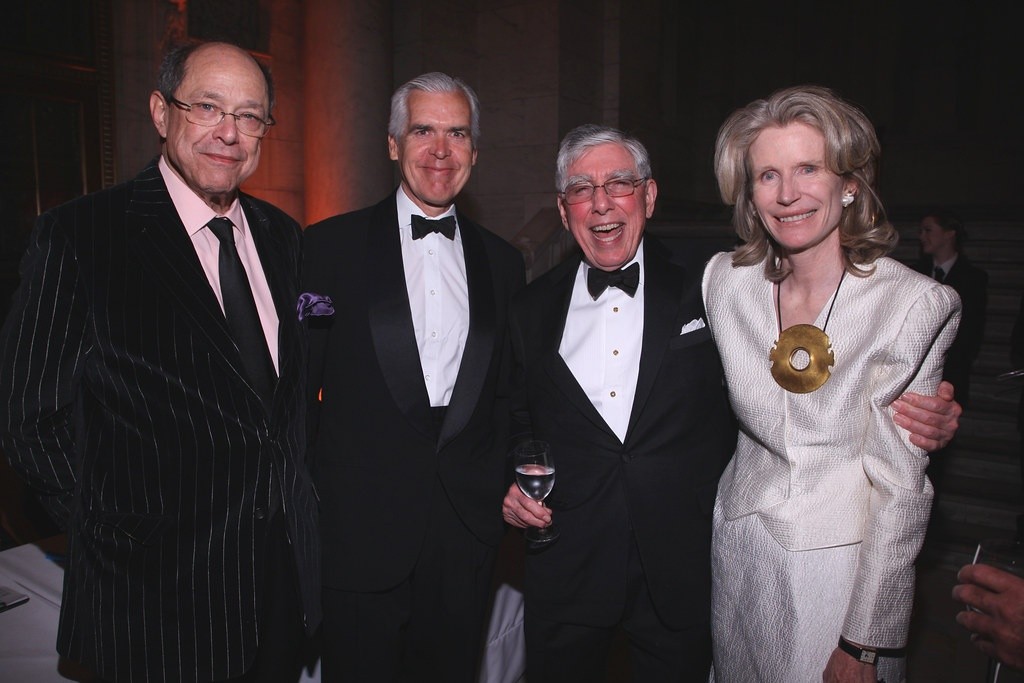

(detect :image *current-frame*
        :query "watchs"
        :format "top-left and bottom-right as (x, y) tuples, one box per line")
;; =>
(837, 635), (879, 665)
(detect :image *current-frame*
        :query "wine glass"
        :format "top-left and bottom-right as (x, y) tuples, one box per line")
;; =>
(966, 540), (1024, 683)
(514, 441), (560, 542)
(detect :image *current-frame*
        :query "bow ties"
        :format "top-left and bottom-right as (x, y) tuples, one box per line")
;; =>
(588, 263), (639, 301)
(412, 215), (456, 241)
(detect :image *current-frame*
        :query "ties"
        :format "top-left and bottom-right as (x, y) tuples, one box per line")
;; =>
(934, 267), (945, 284)
(208, 216), (279, 401)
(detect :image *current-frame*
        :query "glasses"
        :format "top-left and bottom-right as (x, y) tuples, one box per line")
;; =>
(562, 177), (650, 205)
(171, 96), (277, 139)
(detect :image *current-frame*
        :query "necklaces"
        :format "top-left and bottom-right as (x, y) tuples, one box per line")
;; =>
(769, 261), (847, 395)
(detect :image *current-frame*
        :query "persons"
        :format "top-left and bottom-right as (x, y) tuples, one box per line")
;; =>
(0, 41), (326, 683)
(704, 84), (964, 683)
(908, 205), (989, 408)
(953, 564), (1024, 674)
(304, 71), (527, 683)
(502, 124), (963, 683)
